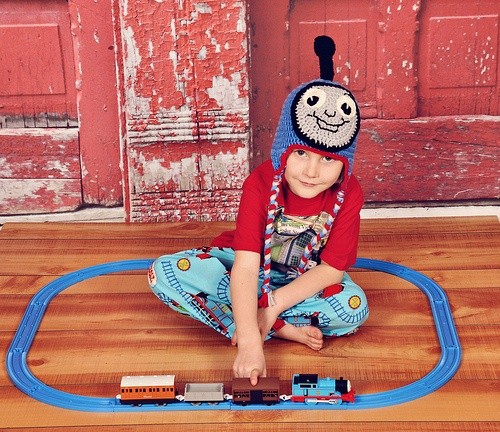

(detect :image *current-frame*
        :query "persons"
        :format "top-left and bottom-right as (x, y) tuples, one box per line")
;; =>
(146, 79), (371, 387)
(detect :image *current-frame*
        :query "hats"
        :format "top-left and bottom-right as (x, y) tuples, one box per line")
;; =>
(257, 77), (363, 309)
